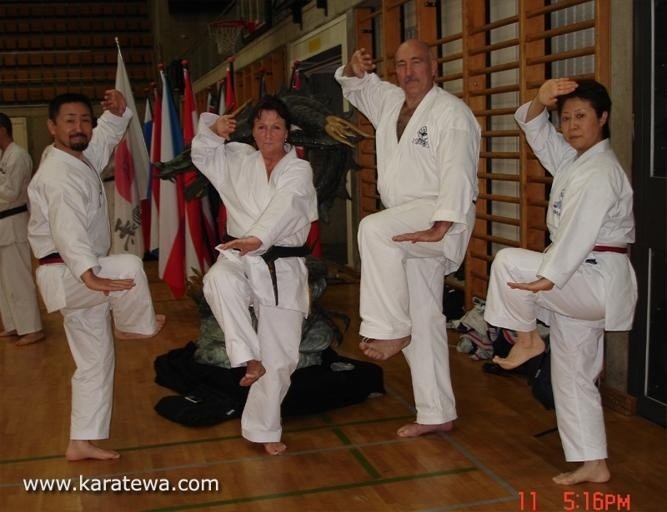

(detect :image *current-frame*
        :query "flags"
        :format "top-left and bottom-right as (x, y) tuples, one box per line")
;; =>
(138, 45), (320, 305)
(110, 34), (152, 267)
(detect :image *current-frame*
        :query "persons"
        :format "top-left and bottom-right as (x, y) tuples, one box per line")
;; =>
(0, 106), (48, 350)
(188, 97), (322, 458)
(24, 87), (167, 463)
(330, 35), (481, 443)
(481, 74), (638, 490)
